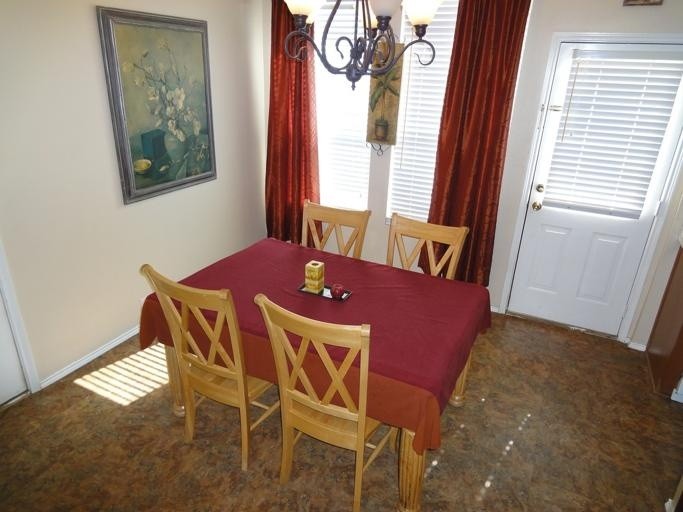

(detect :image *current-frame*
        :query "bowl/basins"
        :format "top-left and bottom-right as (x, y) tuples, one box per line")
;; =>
(132, 158), (151, 175)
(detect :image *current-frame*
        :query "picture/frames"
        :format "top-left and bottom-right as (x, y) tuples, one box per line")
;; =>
(97, 3), (219, 205)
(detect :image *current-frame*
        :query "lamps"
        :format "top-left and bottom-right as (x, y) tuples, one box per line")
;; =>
(279, 0), (447, 98)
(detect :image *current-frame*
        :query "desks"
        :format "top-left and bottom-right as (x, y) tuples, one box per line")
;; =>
(133, 236), (493, 510)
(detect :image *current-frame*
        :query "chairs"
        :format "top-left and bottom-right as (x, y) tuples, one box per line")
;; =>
(296, 195), (374, 259)
(384, 211), (472, 284)
(251, 290), (400, 511)
(136, 258), (275, 474)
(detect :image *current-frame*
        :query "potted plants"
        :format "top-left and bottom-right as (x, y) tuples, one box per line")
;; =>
(368, 55), (400, 141)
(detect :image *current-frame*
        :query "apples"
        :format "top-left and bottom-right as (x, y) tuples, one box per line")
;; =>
(330, 283), (344, 297)
(142, 162), (151, 169)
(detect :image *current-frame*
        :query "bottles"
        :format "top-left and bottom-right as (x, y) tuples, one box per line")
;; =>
(184, 135), (204, 177)
